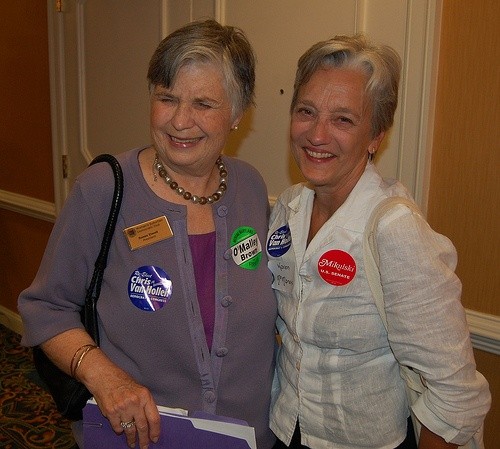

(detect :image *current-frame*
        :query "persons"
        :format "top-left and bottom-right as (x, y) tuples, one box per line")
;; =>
(17, 18), (274, 449)
(267, 31), (494, 448)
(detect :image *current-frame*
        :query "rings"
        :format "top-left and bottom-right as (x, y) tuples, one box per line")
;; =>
(120, 420), (136, 429)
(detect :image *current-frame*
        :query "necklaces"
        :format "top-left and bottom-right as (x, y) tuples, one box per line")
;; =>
(152, 151), (228, 206)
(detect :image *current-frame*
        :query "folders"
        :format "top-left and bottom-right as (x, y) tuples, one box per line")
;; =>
(82, 396), (257, 449)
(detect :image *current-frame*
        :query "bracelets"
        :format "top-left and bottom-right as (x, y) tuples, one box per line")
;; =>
(70, 344), (100, 380)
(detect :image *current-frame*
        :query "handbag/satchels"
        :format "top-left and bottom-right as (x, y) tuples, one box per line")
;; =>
(399, 364), (485, 449)
(32, 304), (99, 421)
